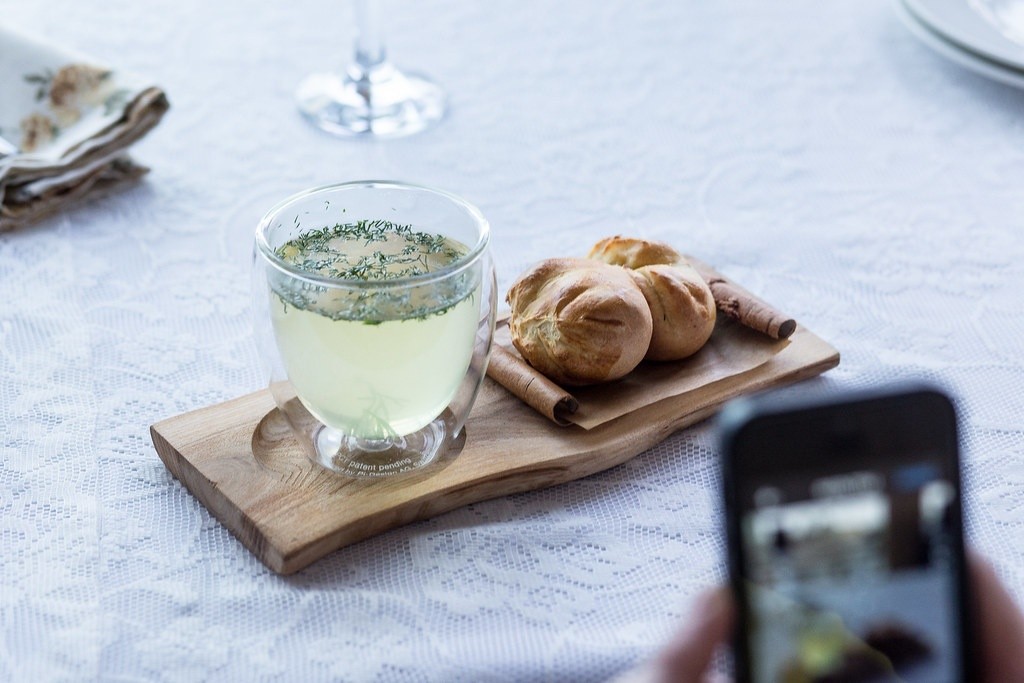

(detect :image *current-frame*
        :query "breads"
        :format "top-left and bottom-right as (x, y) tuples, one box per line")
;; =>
(585, 235), (718, 361)
(504, 255), (653, 384)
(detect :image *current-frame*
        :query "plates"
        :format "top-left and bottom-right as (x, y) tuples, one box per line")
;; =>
(895, 1), (1024, 88)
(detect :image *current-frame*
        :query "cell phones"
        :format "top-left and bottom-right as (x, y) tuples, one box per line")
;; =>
(718, 376), (981, 683)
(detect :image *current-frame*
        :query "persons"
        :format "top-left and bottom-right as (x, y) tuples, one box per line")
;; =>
(613, 545), (1024, 683)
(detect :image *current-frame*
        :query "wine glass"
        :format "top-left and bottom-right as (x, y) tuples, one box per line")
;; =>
(299, 2), (448, 142)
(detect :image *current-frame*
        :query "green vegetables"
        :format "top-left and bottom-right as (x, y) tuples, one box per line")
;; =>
(265, 220), (482, 323)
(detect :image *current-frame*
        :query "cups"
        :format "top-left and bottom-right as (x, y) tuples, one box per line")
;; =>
(253, 180), (498, 481)
